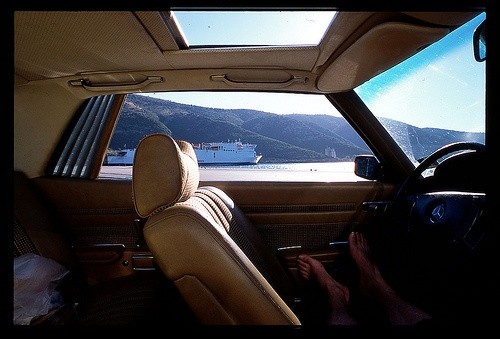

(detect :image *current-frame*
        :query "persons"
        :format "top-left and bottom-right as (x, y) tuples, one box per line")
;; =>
(296, 232), (449, 328)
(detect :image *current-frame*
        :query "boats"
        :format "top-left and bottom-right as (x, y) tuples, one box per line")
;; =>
(107, 138), (263, 166)
